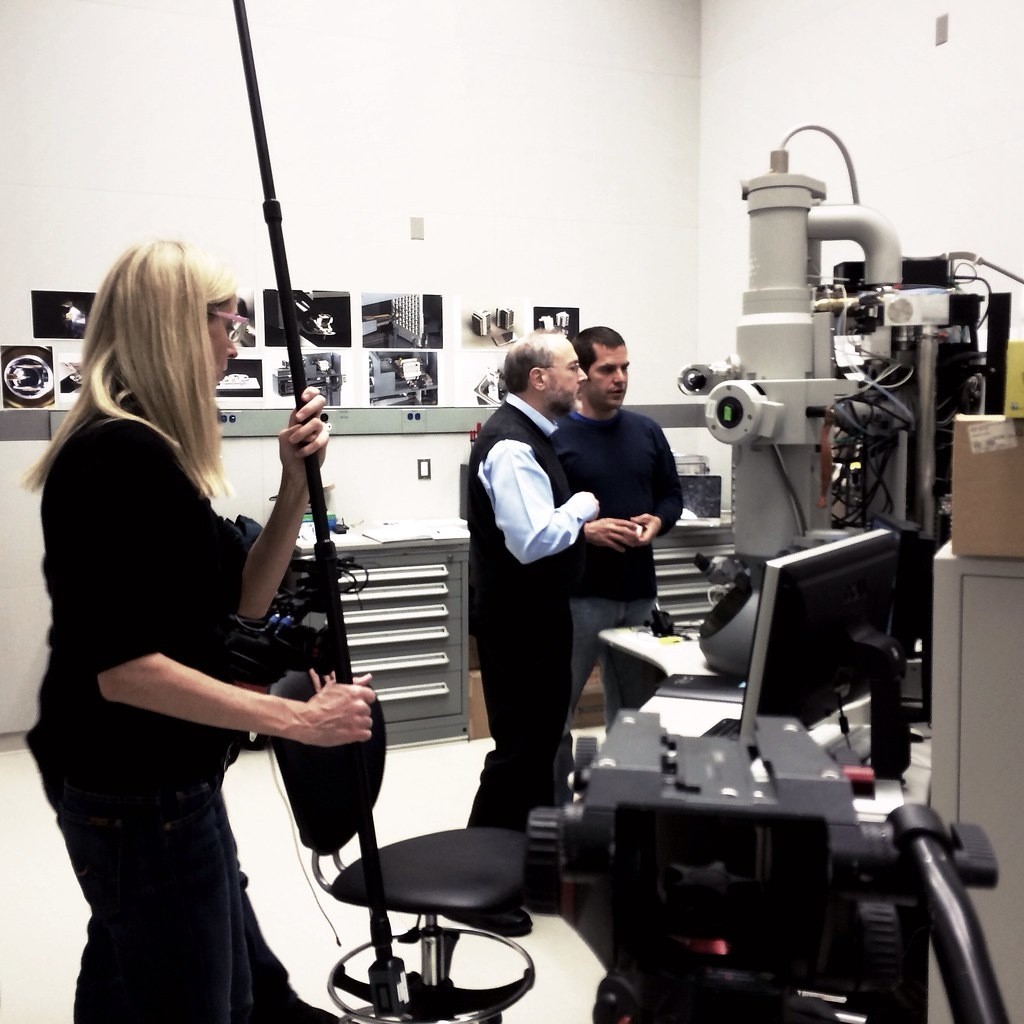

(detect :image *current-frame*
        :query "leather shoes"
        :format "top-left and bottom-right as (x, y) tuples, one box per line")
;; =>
(441, 908), (532, 937)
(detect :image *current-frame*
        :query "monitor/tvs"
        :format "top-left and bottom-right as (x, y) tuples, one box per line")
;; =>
(738, 528), (913, 783)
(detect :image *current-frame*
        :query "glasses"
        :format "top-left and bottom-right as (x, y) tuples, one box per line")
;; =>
(208, 309), (249, 342)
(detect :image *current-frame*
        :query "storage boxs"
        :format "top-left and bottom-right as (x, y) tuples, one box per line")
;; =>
(952, 413), (1024, 555)
(466, 639), (608, 739)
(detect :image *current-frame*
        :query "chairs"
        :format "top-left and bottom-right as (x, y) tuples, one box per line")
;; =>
(270, 666), (536, 1023)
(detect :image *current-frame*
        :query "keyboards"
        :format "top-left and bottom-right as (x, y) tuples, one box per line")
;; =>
(699, 718), (742, 743)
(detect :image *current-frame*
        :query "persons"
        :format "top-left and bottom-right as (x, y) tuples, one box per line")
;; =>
(550, 329), (686, 815)
(22, 242), (377, 1024)
(438, 325), (600, 935)
